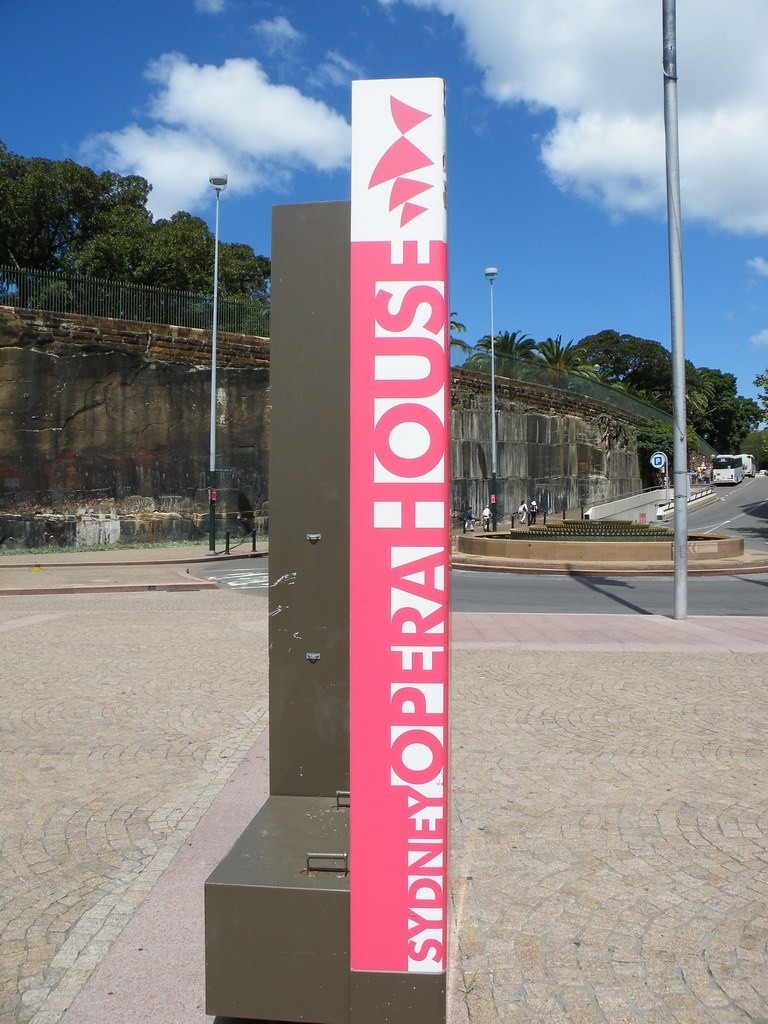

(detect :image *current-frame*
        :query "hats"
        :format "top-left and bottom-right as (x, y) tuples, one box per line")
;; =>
(531, 501), (536, 505)
(486, 505), (489, 507)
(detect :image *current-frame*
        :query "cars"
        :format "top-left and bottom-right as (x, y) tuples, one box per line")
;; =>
(759, 470), (768, 476)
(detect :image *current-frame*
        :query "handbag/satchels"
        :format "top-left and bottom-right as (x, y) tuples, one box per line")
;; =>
(523, 506), (526, 511)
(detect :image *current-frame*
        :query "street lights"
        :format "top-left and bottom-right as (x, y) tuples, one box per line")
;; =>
(207, 170), (228, 556)
(485, 267), (499, 532)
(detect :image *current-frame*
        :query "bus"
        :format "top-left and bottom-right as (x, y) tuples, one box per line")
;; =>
(739, 453), (757, 478)
(711, 454), (747, 487)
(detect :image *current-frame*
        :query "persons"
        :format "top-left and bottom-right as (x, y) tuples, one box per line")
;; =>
(483, 505), (491, 532)
(530, 500), (538, 524)
(465, 507), (474, 531)
(519, 500), (528, 523)
(692, 467), (711, 484)
